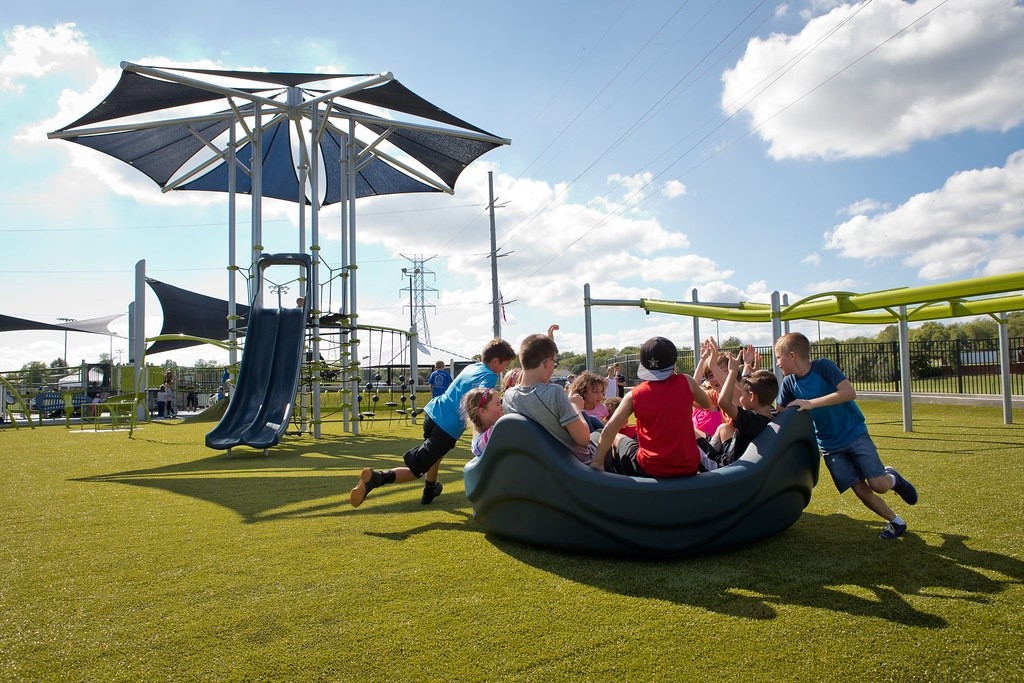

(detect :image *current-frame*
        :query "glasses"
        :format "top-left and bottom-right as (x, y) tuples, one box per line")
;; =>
(552, 359), (560, 370)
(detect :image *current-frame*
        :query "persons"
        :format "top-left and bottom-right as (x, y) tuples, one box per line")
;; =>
(297, 298), (304, 309)
(90, 394), (106, 416)
(428, 362), (453, 398)
(165, 383), (176, 418)
(459, 325), (779, 478)
(350, 337), (516, 508)
(157, 386), (167, 419)
(208, 368), (230, 405)
(774, 333), (918, 540)
(186, 388), (198, 412)
(164, 372), (173, 386)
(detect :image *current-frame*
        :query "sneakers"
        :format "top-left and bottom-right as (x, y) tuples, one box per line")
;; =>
(885, 465), (918, 505)
(350, 467), (383, 508)
(879, 522), (907, 540)
(420, 482), (443, 505)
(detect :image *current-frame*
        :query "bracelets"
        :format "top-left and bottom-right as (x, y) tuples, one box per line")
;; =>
(701, 356), (705, 359)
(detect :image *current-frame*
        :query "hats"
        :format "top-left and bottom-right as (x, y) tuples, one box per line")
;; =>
(637, 337), (677, 381)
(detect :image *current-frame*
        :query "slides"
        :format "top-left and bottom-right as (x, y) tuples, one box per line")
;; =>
(205, 302), (308, 459)
